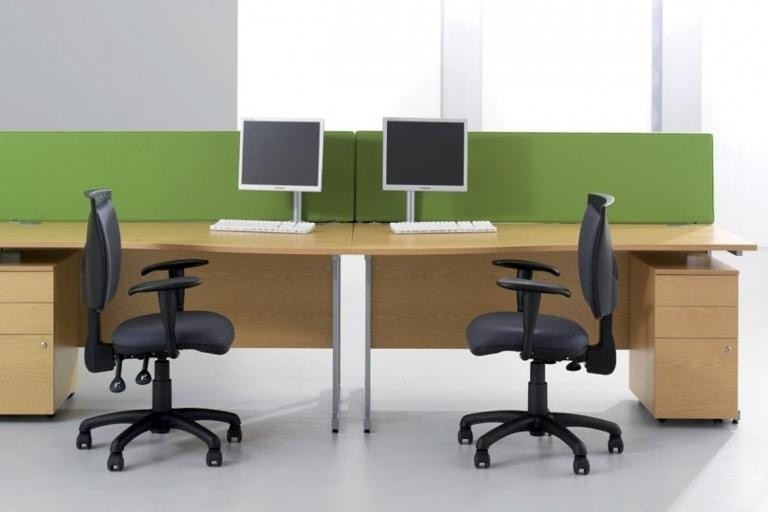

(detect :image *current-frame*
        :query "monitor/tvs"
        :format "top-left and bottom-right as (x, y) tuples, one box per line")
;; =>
(383, 117), (469, 193)
(238, 119), (324, 193)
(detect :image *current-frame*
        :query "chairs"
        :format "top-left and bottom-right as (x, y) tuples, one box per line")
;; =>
(463, 193), (624, 473)
(75, 189), (243, 467)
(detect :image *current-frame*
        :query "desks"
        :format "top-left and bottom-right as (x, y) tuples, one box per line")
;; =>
(355, 132), (758, 434)
(0, 131), (356, 426)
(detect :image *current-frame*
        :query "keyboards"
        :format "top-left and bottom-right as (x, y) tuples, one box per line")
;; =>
(210, 218), (316, 235)
(389, 219), (498, 234)
